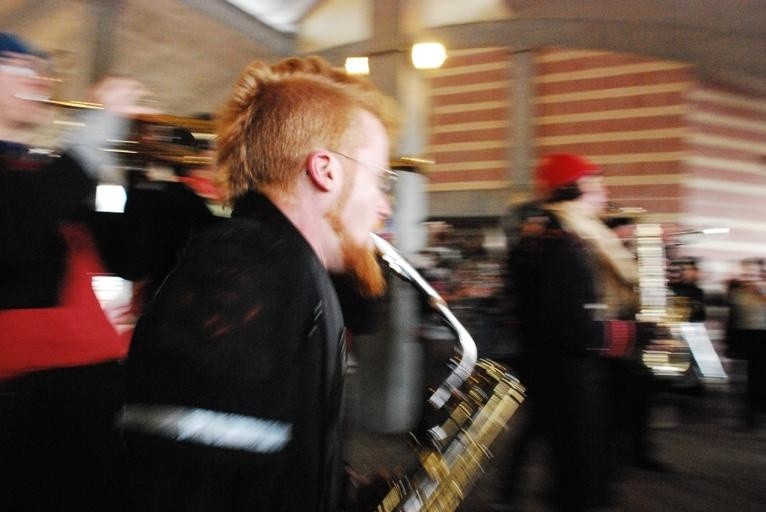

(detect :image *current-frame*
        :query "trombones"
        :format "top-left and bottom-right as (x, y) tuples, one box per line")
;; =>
(24, 97), (438, 186)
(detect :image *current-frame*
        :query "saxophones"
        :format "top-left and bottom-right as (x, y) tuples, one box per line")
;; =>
(366, 230), (527, 512)
(606, 205), (695, 378)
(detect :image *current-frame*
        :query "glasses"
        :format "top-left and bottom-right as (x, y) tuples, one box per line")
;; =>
(305, 149), (400, 193)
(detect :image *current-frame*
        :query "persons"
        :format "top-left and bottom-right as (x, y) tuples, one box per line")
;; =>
(499, 150), (671, 511)
(108, 58), (399, 512)
(602, 215), (764, 470)
(426, 208), (544, 364)
(0, 31), (230, 512)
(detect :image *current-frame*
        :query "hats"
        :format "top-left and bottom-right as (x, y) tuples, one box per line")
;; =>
(534, 154), (602, 186)
